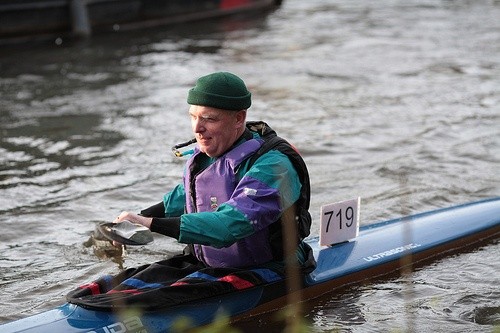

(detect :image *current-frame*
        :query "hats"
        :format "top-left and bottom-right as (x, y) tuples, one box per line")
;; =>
(187, 71), (253, 110)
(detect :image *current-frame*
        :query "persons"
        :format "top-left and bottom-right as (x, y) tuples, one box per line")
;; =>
(110, 70), (306, 270)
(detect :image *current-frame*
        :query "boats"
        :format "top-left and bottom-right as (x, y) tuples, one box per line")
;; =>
(1, 195), (499, 333)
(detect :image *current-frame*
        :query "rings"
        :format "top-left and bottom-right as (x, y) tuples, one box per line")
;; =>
(116, 216), (119, 219)
